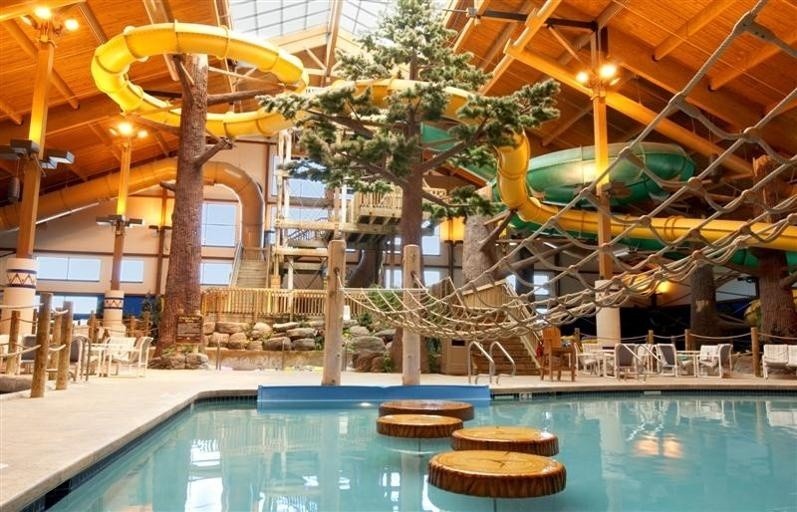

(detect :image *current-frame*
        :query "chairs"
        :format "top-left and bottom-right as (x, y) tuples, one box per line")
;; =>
(0, 323), (156, 383)
(622, 397), (797, 442)
(539, 326), (797, 382)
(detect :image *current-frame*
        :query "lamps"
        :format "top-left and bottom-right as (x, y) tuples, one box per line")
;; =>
(8, 160), (21, 203)
(21, 15), (42, 31)
